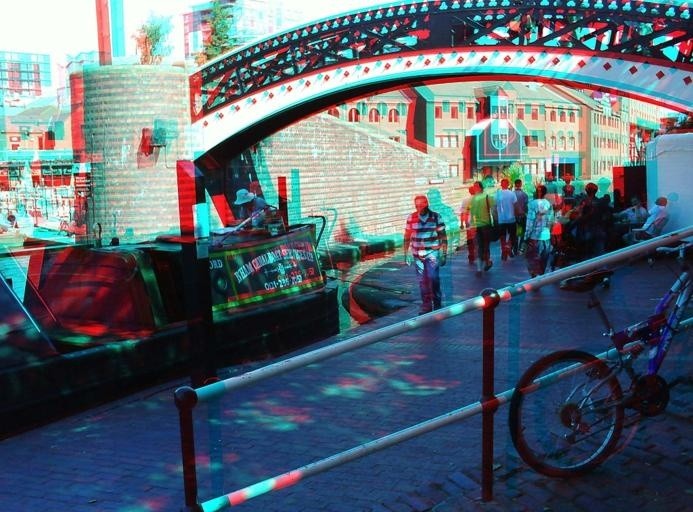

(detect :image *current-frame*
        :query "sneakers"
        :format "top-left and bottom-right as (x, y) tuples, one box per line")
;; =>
(476, 259), (492, 276)
(501, 245), (518, 261)
(417, 309), (427, 314)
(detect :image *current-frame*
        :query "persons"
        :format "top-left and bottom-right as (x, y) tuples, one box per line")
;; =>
(234, 188), (272, 226)
(399, 196), (448, 315)
(458, 171), (670, 279)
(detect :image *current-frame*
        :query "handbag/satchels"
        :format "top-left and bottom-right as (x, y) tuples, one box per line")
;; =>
(491, 227), (499, 241)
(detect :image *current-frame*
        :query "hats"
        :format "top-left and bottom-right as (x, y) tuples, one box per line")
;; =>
(234, 188), (255, 205)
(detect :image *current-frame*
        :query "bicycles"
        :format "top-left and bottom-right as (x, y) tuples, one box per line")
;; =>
(509, 243), (692, 480)
